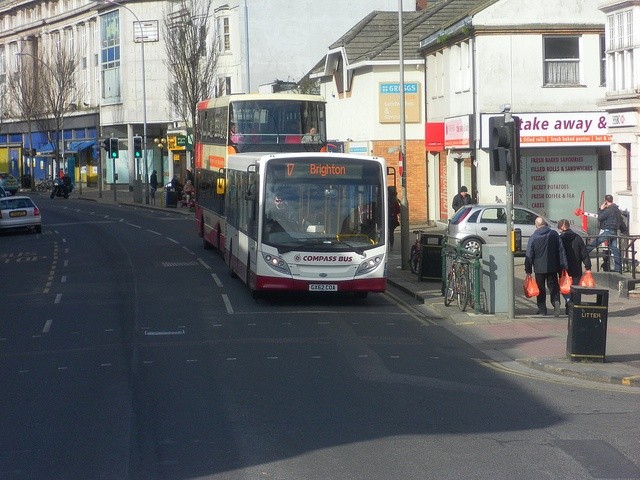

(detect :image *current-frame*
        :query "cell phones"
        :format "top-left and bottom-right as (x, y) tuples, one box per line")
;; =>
(604, 202), (608, 205)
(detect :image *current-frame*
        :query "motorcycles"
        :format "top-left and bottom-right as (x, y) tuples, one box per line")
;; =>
(50, 179), (74, 199)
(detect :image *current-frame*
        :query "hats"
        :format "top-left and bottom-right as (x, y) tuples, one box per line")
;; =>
(459, 186), (467, 192)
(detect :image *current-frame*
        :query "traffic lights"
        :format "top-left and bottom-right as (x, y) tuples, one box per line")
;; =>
(111, 139), (118, 158)
(134, 138), (142, 158)
(104, 138), (109, 151)
(490, 117), (520, 186)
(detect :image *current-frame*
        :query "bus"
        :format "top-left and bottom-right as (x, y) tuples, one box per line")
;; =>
(228, 151), (388, 302)
(196, 95), (327, 253)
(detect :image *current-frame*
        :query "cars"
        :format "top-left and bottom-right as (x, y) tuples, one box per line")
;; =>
(449, 205), (591, 258)
(0, 172), (19, 195)
(0, 196), (41, 232)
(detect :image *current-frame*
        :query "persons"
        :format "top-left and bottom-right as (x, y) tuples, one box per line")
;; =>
(524, 216), (569, 317)
(452, 186), (472, 213)
(585, 195), (622, 275)
(171, 175), (183, 199)
(62, 173), (71, 200)
(149, 169), (158, 199)
(268, 195), (289, 229)
(299, 126), (324, 145)
(390, 191), (401, 246)
(287, 122), (299, 134)
(183, 180), (195, 207)
(557, 218), (592, 316)
(186, 169), (195, 186)
(230, 124), (245, 144)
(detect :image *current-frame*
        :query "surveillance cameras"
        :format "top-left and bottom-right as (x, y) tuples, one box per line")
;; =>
(498, 104), (514, 123)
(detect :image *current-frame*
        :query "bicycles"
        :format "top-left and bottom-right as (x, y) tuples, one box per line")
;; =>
(410, 230), (423, 273)
(445, 254), (469, 312)
(36, 178), (51, 191)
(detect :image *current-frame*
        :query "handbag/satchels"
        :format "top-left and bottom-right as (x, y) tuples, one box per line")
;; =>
(523, 272), (540, 298)
(559, 270), (573, 295)
(620, 210), (629, 234)
(579, 270), (595, 287)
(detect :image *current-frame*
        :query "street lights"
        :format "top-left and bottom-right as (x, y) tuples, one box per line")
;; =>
(154, 130), (167, 187)
(16, 52), (66, 173)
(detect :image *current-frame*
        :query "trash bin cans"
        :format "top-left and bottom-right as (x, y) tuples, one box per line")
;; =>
(165, 185), (178, 209)
(566, 283), (608, 364)
(21, 173), (32, 189)
(415, 234), (446, 283)
